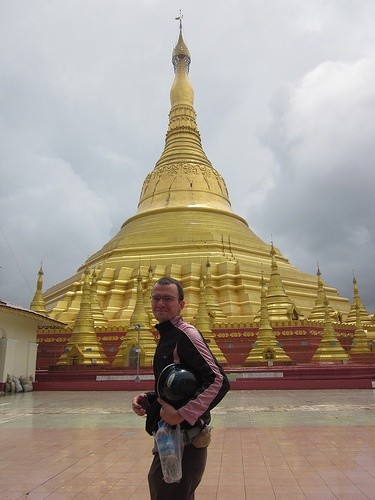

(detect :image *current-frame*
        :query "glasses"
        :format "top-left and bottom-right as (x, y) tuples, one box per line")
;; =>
(150, 296), (178, 302)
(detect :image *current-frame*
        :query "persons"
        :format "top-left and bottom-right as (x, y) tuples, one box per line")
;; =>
(130, 275), (231, 500)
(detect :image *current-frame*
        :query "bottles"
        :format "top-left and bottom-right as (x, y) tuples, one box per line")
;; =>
(154, 420), (181, 484)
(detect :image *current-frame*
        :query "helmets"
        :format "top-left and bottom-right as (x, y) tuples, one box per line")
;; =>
(156, 362), (197, 408)
